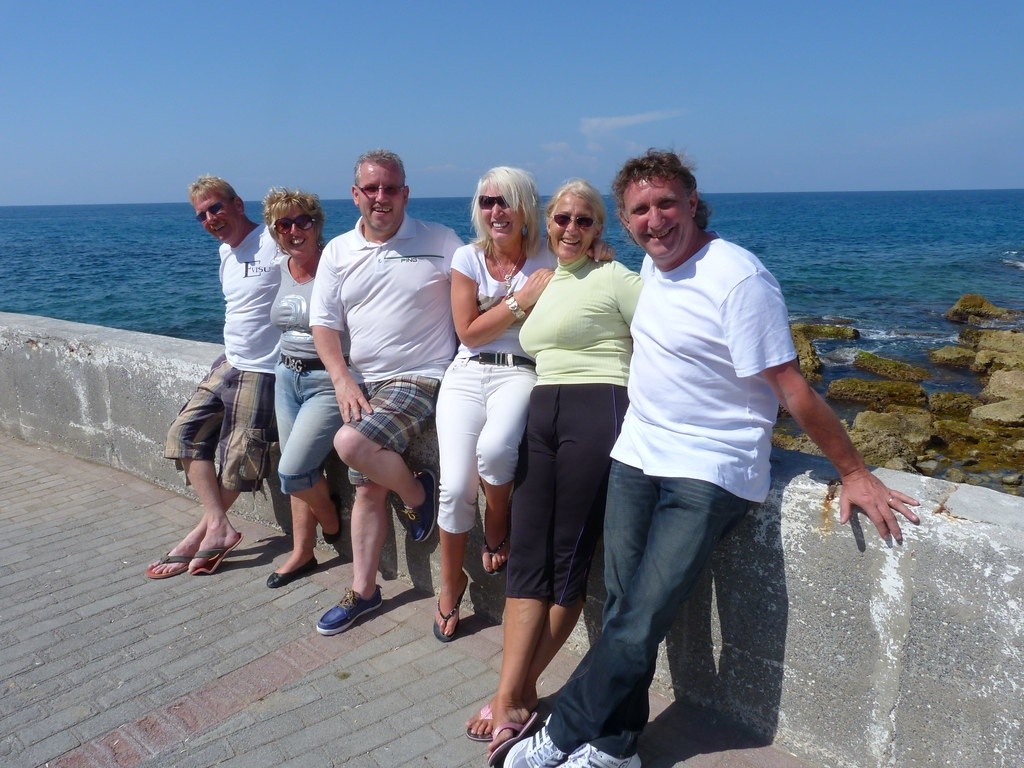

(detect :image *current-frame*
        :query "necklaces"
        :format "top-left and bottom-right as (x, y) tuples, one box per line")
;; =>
(492, 246), (523, 290)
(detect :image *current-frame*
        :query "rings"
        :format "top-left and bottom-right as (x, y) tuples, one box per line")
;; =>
(887, 496), (894, 505)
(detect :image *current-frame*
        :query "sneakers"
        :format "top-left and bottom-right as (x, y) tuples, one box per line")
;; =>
(503, 713), (568, 768)
(316, 584), (382, 636)
(555, 741), (641, 768)
(400, 468), (438, 543)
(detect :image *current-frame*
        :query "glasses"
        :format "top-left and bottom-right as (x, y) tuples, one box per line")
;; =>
(478, 195), (510, 210)
(550, 214), (597, 228)
(355, 184), (406, 198)
(271, 213), (316, 235)
(195, 197), (234, 223)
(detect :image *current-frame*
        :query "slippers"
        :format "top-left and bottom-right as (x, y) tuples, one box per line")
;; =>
(487, 710), (539, 768)
(146, 550), (194, 579)
(433, 574), (469, 643)
(466, 702), (540, 742)
(482, 499), (512, 576)
(191, 531), (245, 575)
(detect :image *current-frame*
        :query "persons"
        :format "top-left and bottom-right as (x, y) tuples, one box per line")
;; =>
(262, 186), (345, 587)
(146, 175), (288, 579)
(502, 152), (920, 768)
(433, 168), (615, 642)
(309, 150), (466, 635)
(466, 180), (644, 767)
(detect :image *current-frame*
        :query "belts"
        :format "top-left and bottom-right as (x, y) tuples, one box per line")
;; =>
(464, 351), (536, 366)
(279, 352), (350, 373)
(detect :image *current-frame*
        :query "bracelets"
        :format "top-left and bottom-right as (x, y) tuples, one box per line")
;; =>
(505, 296), (525, 320)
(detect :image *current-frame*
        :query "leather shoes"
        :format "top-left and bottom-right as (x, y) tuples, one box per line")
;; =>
(321, 493), (342, 544)
(266, 555), (319, 589)
